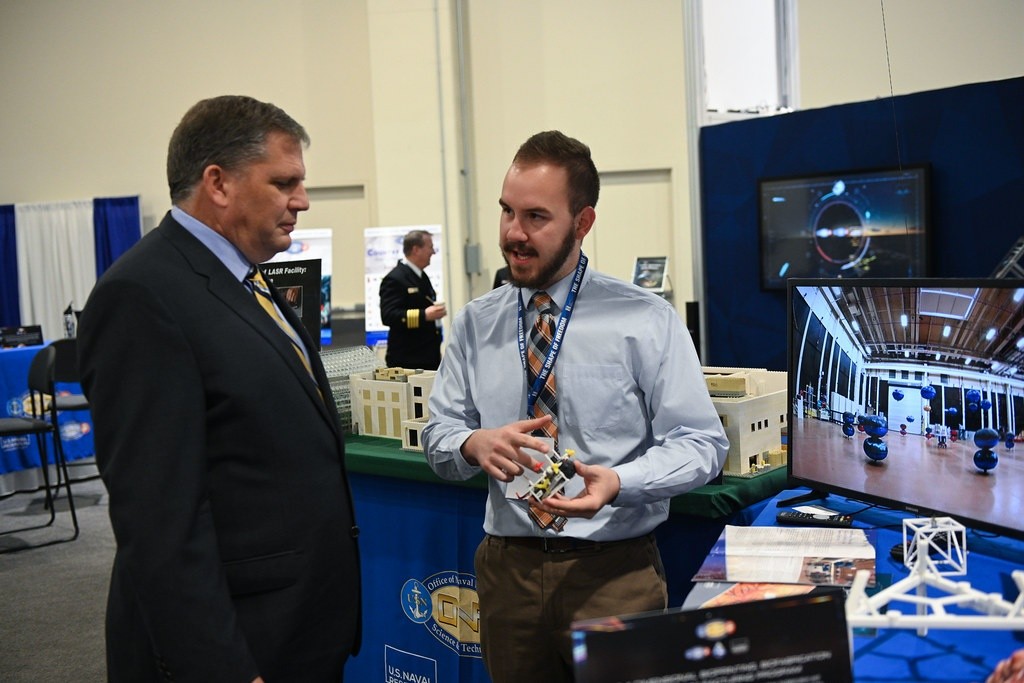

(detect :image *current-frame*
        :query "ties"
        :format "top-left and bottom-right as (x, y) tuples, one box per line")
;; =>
(244, 263), (324, 410)
(528, 291), (568, 531)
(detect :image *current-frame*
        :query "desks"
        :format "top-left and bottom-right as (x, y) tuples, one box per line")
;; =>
(341, 430), (789, 683)
(680, 485), (1024, 683)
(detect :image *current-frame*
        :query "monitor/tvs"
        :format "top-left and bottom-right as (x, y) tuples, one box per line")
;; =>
(786, 277), (1024, 542)
(558, 585), (857, 683)
(755, 162), (933, 292)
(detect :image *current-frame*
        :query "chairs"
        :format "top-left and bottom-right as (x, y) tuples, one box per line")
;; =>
(0, 346), (79, 554)
(41, 339), (102, 511)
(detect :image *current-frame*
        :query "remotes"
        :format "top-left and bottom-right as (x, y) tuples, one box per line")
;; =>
(891, 530), (965, 563)
(777, 510), (854, 528)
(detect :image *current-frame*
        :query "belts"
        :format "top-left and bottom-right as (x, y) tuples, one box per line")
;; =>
(486, 532), (608, 553)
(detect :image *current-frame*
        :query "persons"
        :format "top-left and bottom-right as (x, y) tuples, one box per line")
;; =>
(420, 130), (731, 683)
(379, 230), (448, 369)
(78, 96), (360, 683)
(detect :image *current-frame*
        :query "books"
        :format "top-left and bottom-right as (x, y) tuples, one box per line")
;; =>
(694, 524), (876, 587)
